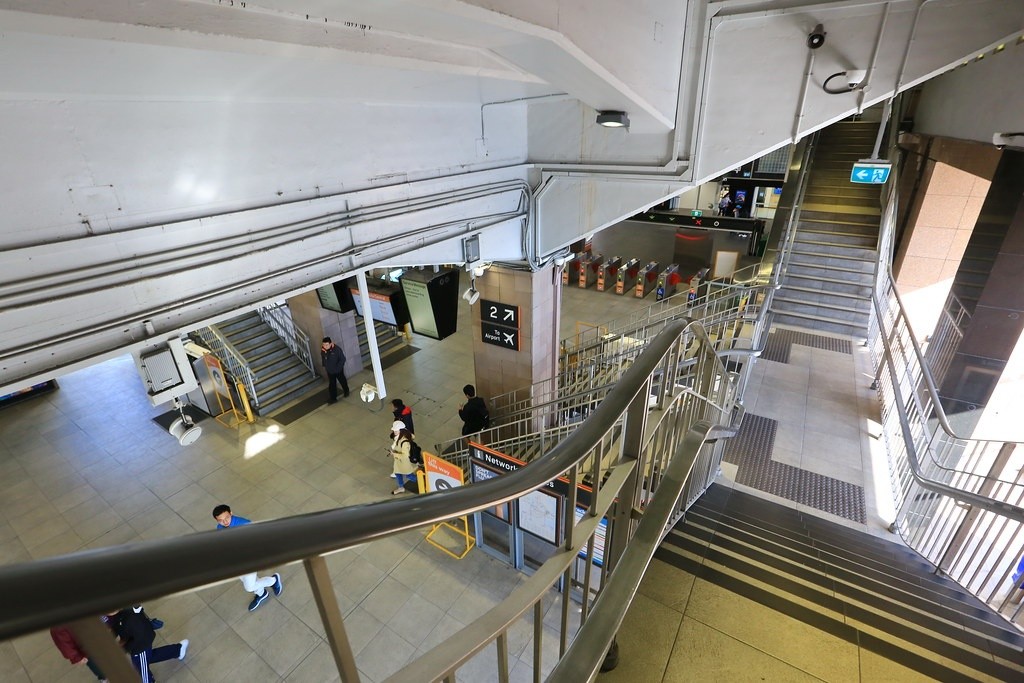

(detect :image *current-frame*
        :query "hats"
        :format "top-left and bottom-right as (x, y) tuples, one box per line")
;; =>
(391, 421), (405, 430)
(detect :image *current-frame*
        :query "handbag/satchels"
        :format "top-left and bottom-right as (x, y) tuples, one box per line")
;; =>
(148, 618), (164, 630)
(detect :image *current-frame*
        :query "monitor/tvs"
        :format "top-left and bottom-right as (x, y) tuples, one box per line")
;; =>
(347, 285), (399, 328)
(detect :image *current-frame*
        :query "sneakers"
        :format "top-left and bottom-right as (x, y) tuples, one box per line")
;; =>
(271, 573), (282, 596)
(248, 589), (269, 612)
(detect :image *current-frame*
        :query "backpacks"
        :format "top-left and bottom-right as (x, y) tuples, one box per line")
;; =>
(467, 398), (489, 432)
(401, 440), (421, 464)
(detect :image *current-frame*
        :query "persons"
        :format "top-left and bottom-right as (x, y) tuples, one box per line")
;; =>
(320, 337), (349, 407)
(390, 420), (418, 495)
(50, 626), (107, 683)
(458, 384), (486, 448)
(99, 603), (188, 683)
(390, 399), (414, 441)
(212, 505), (282, 612)
(717, 193), (731, 216)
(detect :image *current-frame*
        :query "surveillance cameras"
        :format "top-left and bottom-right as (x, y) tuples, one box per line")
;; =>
(845, 70), (866, 89)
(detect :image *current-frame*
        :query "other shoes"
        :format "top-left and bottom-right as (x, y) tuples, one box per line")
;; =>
(391, 487), (406, 495)
(327, 398), (337, 403)
(344, 387), (349, 397)
(177, 639), (189, 660)
(390, 473), (396, 478)
